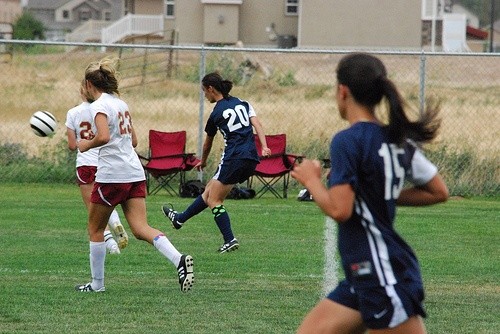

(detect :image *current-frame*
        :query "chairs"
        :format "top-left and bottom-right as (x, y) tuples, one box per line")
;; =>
(138, 130), (200, 197)
(247, 134), (306, 199)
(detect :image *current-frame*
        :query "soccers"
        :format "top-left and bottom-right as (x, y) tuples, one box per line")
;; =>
(29, 110), (58, 138)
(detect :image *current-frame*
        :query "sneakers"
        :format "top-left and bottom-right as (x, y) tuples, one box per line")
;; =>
(216, 238), (240, 254)
(162, 205), (184, 230)
(114, 225), (128, 249)
(75, 283), (106, 292)
(177, 254), (195, 293)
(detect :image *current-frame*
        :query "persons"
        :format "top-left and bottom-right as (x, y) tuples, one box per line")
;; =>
(160, 72), (272, 253)
(291, 52), (448, 334)
(73, 57), (194, 294)
(265, 21), (280, 46)
(420, 25), (430, 47)
(64, 78), (129, 255)
